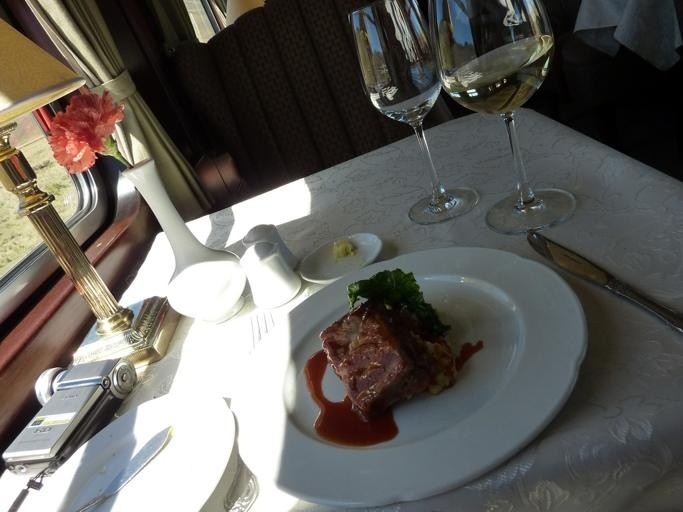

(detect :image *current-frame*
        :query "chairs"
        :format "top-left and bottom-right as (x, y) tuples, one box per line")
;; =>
(173, 0), (453, 193)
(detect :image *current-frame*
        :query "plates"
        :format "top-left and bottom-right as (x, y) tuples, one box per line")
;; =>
(35, 392), (235, 512)
(300, 233), (381, 283)
(236, 247), (588, 506)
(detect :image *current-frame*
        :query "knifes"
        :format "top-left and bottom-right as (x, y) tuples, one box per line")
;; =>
(528, 230), (682, 333)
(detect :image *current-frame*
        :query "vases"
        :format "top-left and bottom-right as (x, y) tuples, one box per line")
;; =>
(113, 160), (251, 328)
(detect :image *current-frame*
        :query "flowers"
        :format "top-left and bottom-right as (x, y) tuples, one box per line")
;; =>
(45, 86), (137, 173)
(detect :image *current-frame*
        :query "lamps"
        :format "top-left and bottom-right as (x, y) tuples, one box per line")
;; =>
(0, 15), (181, 371)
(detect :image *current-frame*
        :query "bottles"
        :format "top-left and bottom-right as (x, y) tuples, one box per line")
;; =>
(240, 223), (301, 308)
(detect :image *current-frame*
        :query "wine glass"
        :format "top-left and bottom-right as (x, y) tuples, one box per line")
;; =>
(349, 0), (480, 225)
(428, 0), (577, 233)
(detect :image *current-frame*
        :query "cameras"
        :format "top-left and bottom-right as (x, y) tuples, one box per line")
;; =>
(0, 357), (136, 476)
